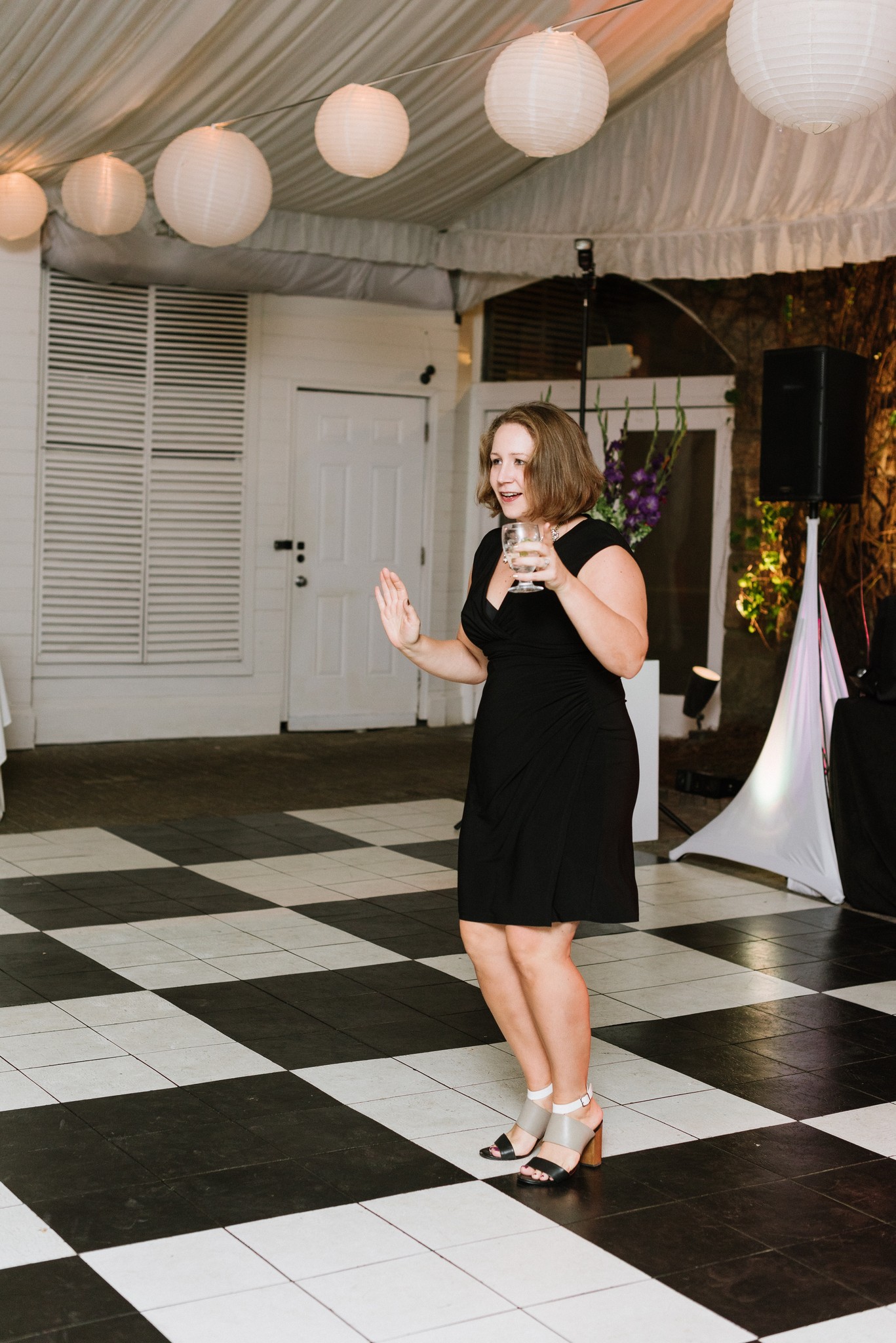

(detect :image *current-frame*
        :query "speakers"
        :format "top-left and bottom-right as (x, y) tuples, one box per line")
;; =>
(757, 345), (870, 505)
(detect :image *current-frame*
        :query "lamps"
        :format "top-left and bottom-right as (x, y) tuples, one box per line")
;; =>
(680, 662), (723, 730)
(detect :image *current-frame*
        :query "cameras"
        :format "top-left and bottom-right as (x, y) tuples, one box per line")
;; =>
(574, 239), (595, 272)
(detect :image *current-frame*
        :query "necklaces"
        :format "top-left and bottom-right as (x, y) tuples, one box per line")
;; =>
(502, 520), (567, 569)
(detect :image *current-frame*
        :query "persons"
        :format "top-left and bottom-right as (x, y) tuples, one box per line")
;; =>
(371, 396), (651, 1192)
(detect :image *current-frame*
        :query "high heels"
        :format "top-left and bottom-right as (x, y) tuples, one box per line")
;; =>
(478, 1072), (552, 1164)
(518, 1085), (606, 1184)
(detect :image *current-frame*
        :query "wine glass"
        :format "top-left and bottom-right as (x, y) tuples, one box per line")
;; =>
(501, 523), (544, 592)
